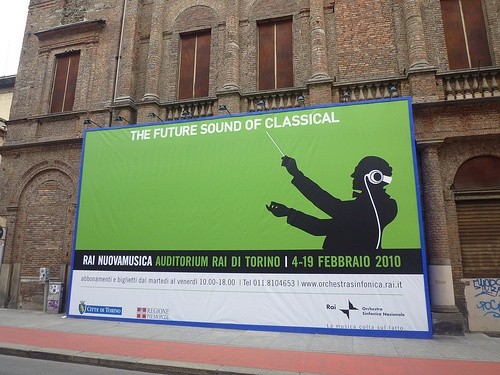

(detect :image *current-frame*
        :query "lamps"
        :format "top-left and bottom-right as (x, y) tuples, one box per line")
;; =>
(182, 110), (195, 119)
(219, 104), (231, 115)
(83, 119), (103, 128)
(115, 115), (132, 124)
(146, 112), (164, 122)
(257, 100), (266, 111)
(343, 91), (350, 101)
(297, 96), (306, 107)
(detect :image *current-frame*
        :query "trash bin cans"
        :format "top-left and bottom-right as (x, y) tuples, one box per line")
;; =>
(46, 281), (65, 314)
